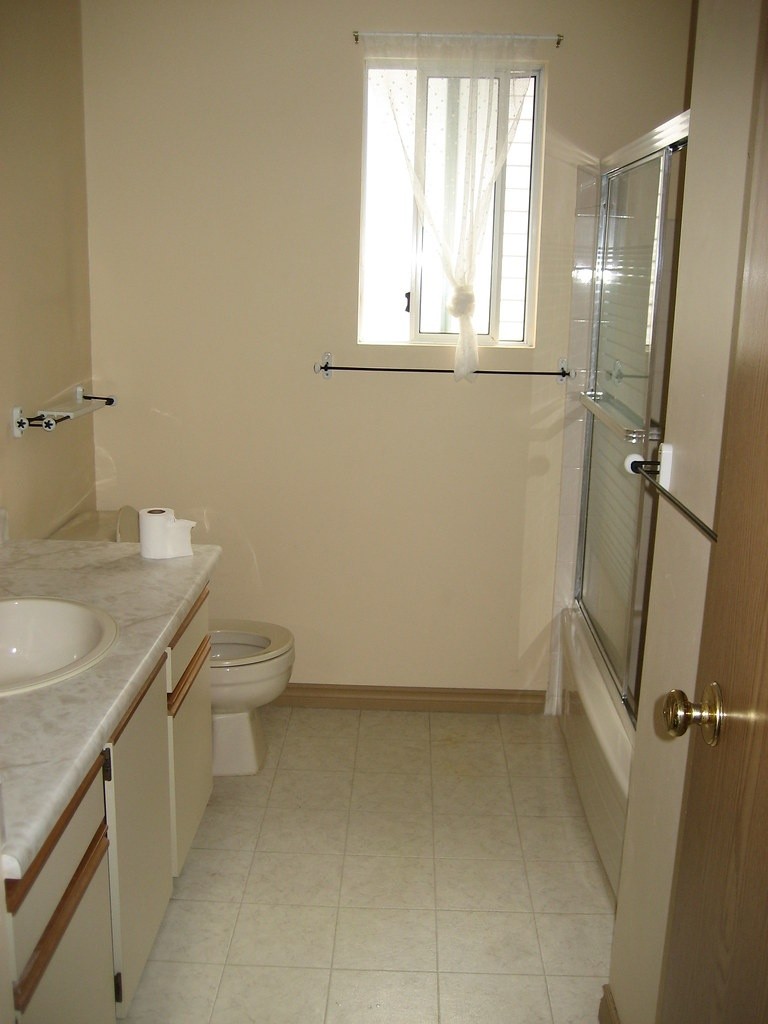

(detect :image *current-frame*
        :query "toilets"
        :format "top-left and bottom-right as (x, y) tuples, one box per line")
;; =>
(43, 505), (295, 776)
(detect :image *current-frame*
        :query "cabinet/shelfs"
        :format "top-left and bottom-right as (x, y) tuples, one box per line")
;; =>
(164, 577), (216, 879)
(101, 651), (174, 1021)
(0, 749), (120, 1024)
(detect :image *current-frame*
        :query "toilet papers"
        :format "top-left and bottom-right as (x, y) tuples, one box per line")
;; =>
(136, 507), (198, 559)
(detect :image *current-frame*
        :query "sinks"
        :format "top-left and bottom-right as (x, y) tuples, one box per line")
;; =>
(0, 595), (118, 698)
